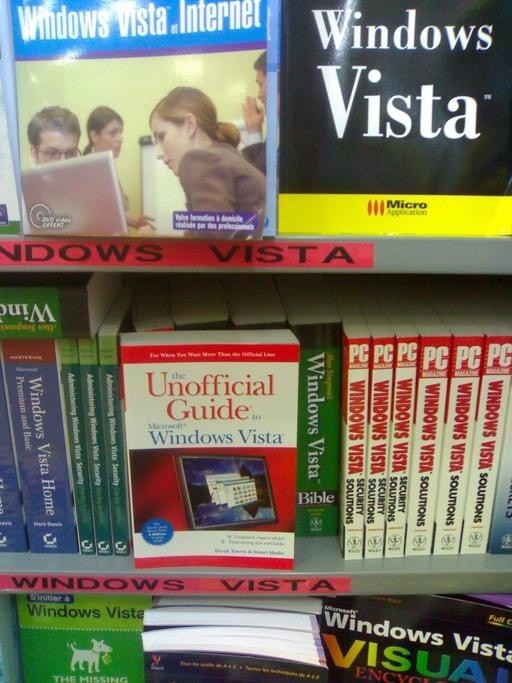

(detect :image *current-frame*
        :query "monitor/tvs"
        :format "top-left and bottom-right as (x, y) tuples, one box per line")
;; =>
(175, 453), (278, 533)
(20, 151), (129, 238)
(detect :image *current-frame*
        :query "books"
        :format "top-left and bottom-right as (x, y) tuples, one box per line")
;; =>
(0, 0), (510, 683)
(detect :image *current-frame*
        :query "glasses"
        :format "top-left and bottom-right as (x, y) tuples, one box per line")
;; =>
(44, 148), (81, 159)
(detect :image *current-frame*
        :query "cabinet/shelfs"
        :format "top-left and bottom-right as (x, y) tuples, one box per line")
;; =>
(0, 233), (512, 682)
